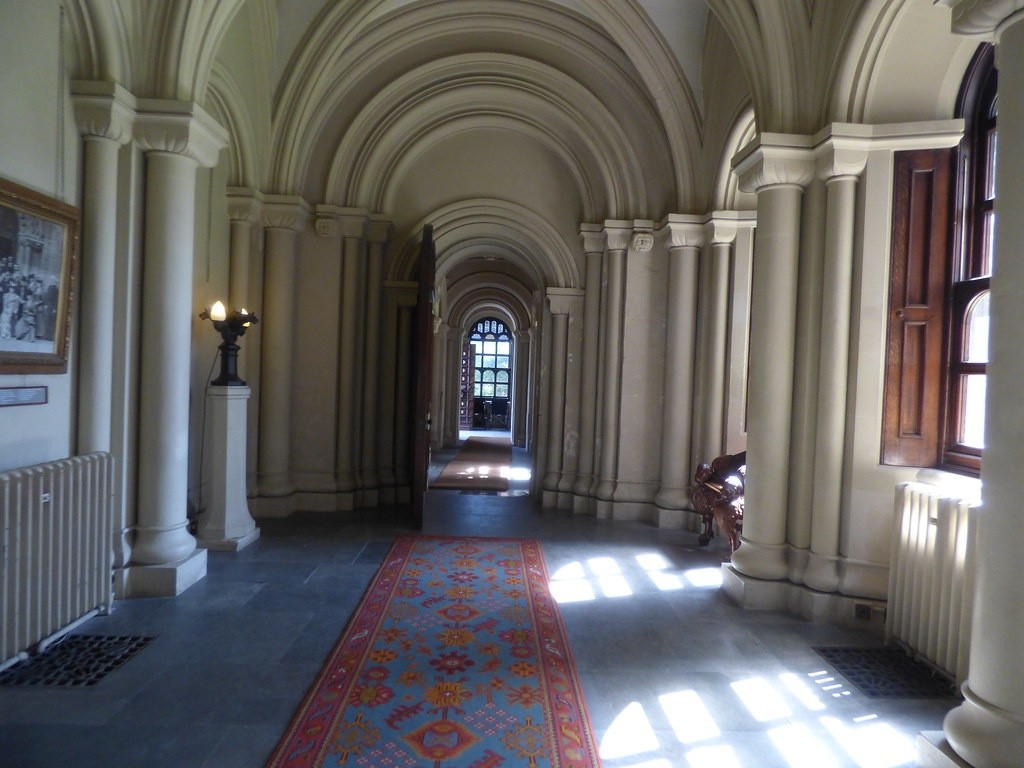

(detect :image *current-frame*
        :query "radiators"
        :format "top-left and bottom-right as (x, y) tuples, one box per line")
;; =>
(883, 480), (981, 686)
(0, 451), (116, 662)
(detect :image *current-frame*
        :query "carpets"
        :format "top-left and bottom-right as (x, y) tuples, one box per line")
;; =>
(0, 633), (161, 690)
(811, 644), (960, 701)
(428, 436), (513, 492)
(272, 533), (604, 768)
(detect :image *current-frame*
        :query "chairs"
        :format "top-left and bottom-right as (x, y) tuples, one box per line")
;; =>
(474, 397), (508, 429)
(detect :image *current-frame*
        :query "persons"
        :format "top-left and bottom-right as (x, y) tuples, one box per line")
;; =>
(0, 256), (49, 341)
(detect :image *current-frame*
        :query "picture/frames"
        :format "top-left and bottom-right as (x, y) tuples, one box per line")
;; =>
(0, 177), (84, 375)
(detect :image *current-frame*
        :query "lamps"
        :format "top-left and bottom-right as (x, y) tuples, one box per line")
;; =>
(198, 300), (259, 386)
